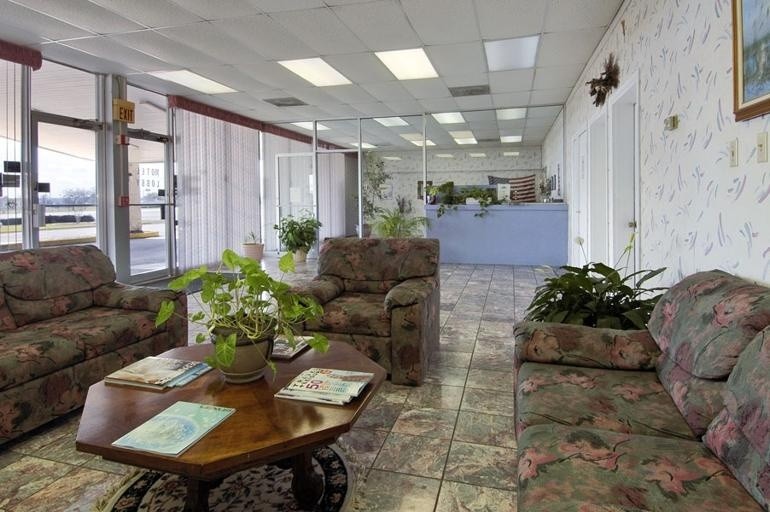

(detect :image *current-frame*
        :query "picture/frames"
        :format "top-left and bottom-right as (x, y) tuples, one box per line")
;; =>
(731, 0), (770, 122)
(550, 163), (560, 196)
(417, 180), (433, 199)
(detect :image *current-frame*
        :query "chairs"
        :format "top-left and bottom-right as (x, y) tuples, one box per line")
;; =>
(288, 237), (439, 386)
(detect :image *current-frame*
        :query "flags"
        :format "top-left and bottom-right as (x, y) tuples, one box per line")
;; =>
(487, 174), (536, 202)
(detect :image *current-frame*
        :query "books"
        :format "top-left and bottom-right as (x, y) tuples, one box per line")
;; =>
(271, 333), (315, 360)
(112, 399), (237, 459)
(102, 355), (214, 391)
(273, 367), (375, 406)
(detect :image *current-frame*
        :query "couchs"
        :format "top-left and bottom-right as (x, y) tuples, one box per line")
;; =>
(513, 269), (770, 512)
(0, 245), (187, 446)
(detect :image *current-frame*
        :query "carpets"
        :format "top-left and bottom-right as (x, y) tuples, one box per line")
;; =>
(92, 442), (365, 512)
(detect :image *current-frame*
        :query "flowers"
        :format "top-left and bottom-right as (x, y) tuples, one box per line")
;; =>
(523, 224), (667, 331)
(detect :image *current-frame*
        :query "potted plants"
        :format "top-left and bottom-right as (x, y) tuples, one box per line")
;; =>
(352, 193), (377, 237)
(155, 249), (330, 385)
(274, 209), (322, 264)
(239, 230), (264, 266)
